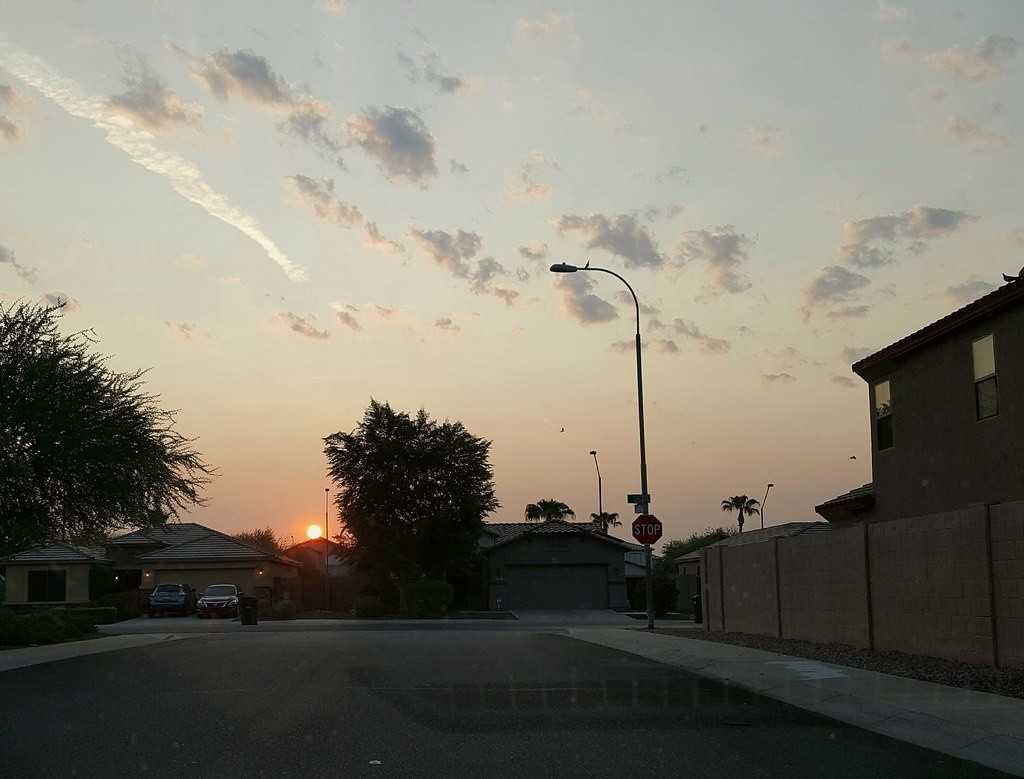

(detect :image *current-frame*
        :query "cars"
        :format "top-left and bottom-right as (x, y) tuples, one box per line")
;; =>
(149, 582), (196, 617)
(196, 584), (243, 619)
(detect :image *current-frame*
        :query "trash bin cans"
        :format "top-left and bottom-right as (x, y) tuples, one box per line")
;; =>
(238, 597), (259, 626)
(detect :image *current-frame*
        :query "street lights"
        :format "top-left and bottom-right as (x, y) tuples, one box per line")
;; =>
(324, 488), (330, 577)
(589, 450), (602, 515)
(761, 483), (774, 528)
(550, 262), (655, 630)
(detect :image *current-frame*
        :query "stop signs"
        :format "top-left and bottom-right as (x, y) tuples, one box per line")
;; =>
(631, 514), (663, 545)
